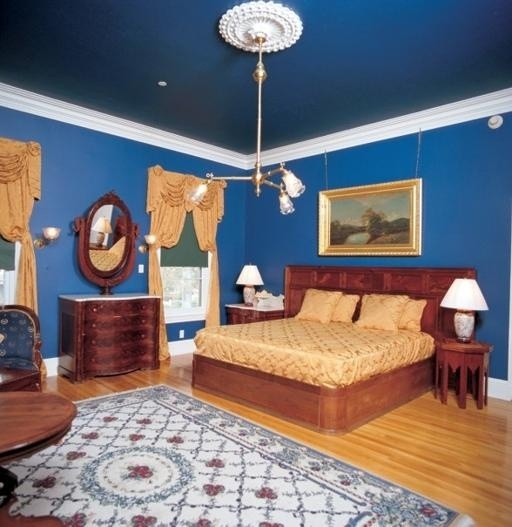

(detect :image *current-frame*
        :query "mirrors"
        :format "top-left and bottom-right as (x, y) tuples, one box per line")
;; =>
(75, 190), (138, 289)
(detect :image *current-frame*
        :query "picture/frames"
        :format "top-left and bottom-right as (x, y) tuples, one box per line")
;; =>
(316, 175), (424, 258)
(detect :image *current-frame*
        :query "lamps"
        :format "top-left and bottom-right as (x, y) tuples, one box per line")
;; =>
(186, 0), (307, 219)
(139, 233), (156, 256)
(235, 261), (265, 304)
(32, 224), (61, 250)
(437, 277), (489, 343)
(91, 214), (113, 248)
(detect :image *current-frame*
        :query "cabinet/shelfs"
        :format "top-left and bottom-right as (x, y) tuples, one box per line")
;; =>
(55, 292), (162, 385)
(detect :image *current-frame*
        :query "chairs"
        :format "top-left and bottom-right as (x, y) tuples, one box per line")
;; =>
(0, 305), (45, 394)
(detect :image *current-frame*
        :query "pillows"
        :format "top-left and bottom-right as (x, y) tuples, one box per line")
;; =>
(293, 285), (427, 335)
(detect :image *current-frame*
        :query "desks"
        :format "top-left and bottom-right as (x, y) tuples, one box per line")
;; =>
(223, 301), (285, 324)
(0, 389), (78, 527)
(431, 338), (494, 410)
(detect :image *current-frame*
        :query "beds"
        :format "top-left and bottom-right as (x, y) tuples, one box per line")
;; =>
(190, 263), (479, 437)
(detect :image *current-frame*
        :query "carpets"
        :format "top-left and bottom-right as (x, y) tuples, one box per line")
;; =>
(2, 382), (481, 526)
